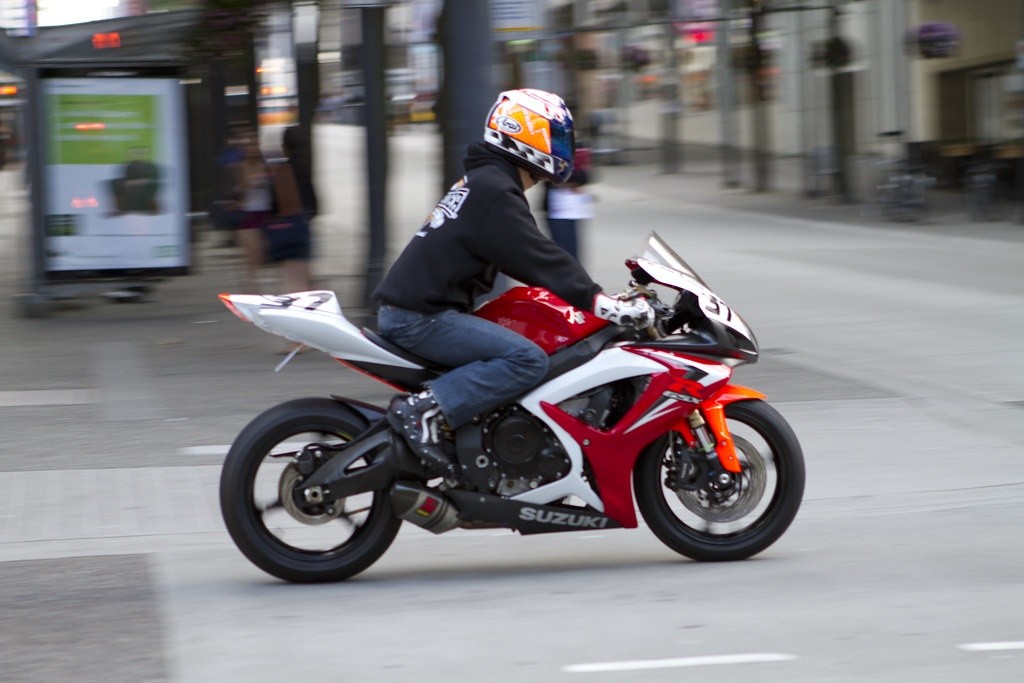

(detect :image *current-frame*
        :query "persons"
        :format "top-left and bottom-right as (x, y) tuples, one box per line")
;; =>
(541, 165), (589, 260)
(208, 127), (320, 293)
(367, 88), (658, 477)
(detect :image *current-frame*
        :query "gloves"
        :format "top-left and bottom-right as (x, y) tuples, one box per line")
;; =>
(591, 291), (655, 331)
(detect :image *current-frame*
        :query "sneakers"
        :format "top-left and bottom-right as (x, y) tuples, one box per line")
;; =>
(385, 389), (455, 480)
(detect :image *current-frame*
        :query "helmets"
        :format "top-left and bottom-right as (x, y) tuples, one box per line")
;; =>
(484, 88), (576, 186)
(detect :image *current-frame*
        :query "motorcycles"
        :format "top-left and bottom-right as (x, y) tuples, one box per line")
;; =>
(218, 228), (805, 584)
